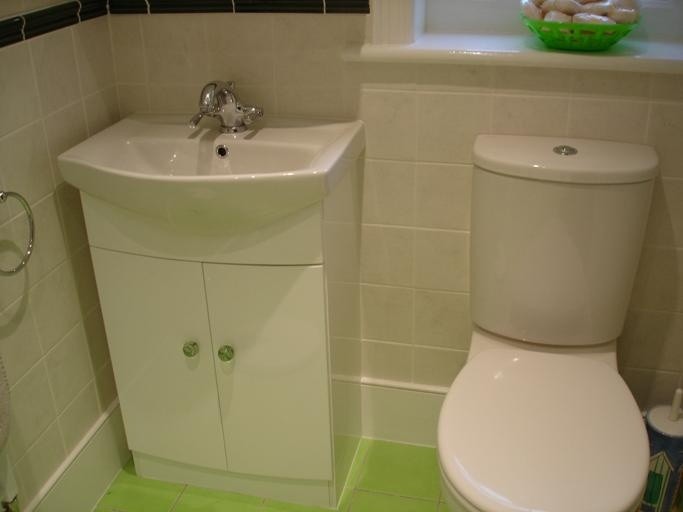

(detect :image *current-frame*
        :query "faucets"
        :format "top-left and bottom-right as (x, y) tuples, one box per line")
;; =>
(188, 80), (265, 133)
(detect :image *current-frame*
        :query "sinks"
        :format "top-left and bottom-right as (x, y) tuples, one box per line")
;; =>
(57, 118), (366, 204)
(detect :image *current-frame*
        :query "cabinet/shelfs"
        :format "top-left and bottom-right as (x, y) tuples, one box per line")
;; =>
(79, 174), (362, 510)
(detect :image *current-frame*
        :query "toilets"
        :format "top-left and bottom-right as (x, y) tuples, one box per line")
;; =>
(438, 128), (658, 511)
